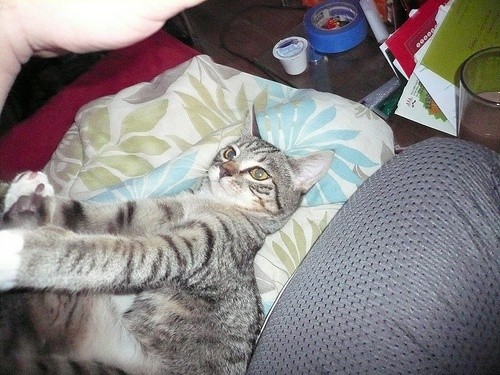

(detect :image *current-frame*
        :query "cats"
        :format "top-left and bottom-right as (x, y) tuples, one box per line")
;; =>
(1, 103), (340, 372)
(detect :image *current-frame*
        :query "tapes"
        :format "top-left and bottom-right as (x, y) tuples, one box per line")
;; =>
(301, 1), (369, 53)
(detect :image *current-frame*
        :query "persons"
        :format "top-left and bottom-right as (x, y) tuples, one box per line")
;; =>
(0, 0), (205, 118)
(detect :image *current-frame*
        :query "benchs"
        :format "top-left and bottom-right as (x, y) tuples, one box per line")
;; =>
(0, 28), (499, 374)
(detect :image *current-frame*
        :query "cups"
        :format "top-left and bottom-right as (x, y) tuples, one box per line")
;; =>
(458, 46), (500, 156)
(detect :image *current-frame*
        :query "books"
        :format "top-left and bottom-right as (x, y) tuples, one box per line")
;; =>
(357, 0), (500, 136)
